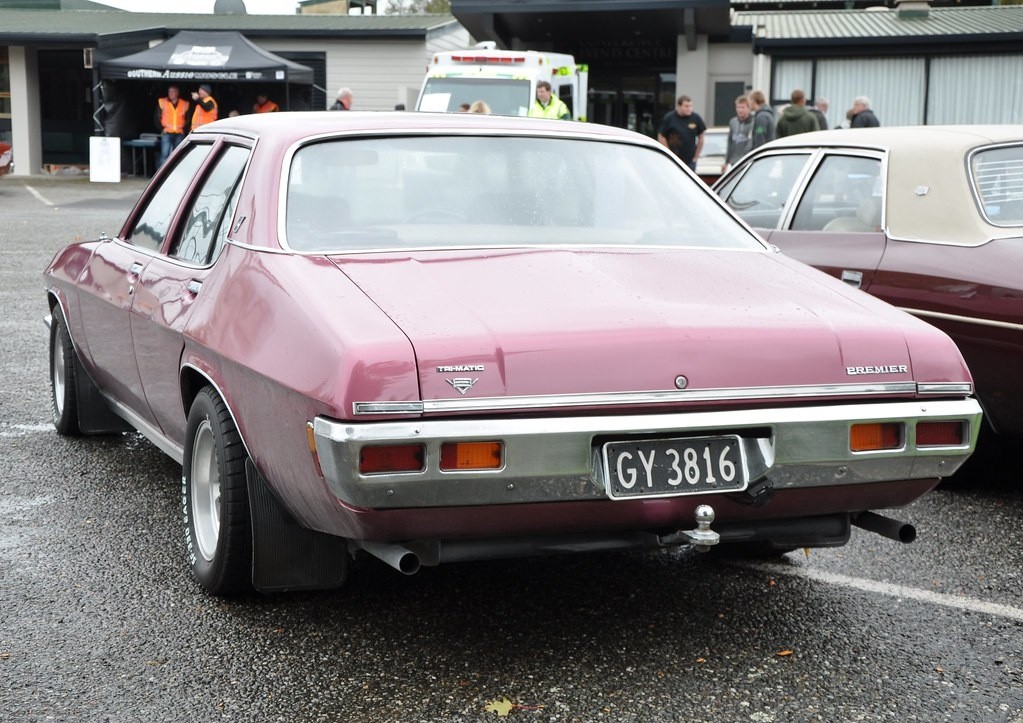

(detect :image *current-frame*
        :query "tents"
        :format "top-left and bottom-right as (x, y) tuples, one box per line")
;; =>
(97, 30), (314, 175)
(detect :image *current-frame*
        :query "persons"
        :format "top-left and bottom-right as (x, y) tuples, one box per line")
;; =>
(330, 88), (353, 111)
(459, 101), (487, 113)
(228, 109), (241, 118)
(157, 86), (190, 165)
(658, 95), (707, 172)
(189, 85), (218, 131)
(529, 82), (571, 121)
(253, 93), (278, 114)
(721, 91), (775, 196)
(832, 97), (881, 206)
(395, 104), (405, 110)
(776, 91), (828, 200)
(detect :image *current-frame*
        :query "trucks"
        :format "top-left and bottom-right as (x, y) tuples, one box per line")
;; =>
(415, 50), (588, 121)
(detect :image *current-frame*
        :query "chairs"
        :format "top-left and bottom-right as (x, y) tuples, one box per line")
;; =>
(822, 196), (881, 231)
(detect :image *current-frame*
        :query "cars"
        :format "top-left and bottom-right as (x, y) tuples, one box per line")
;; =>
(712, 125), (1023, 494)
(45, 110), (984, 596)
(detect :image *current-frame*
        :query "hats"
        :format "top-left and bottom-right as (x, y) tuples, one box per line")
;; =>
(200, 84), (212, 95)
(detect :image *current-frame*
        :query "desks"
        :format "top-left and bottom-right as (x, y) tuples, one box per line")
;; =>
(123, 140), (160, 179)
